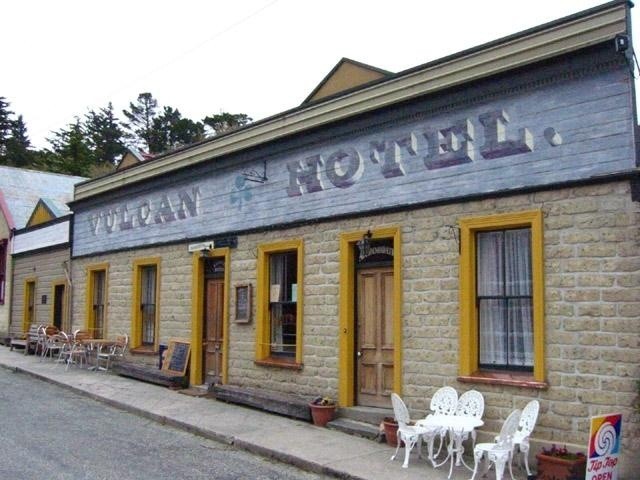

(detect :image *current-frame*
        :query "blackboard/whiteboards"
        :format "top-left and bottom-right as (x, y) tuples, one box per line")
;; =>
(161, 340), (191, 377)
(232, 282), (252, 324)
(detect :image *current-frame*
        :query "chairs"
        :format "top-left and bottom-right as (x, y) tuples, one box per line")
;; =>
(391, 385), (540, 480)
(9, 320), (130, 373)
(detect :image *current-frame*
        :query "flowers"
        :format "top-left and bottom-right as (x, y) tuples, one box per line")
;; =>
(537, 442), (583, 460)
(314, 395), (334, 405)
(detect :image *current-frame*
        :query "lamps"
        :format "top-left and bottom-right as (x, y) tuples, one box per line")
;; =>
(357, 230), (373, 255)
(200, 244), (212, 258)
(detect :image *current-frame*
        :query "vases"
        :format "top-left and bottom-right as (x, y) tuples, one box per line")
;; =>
(307, 401), (338, 426)
(383, 417), (406, 448)
(534, 451), (587, 480)
